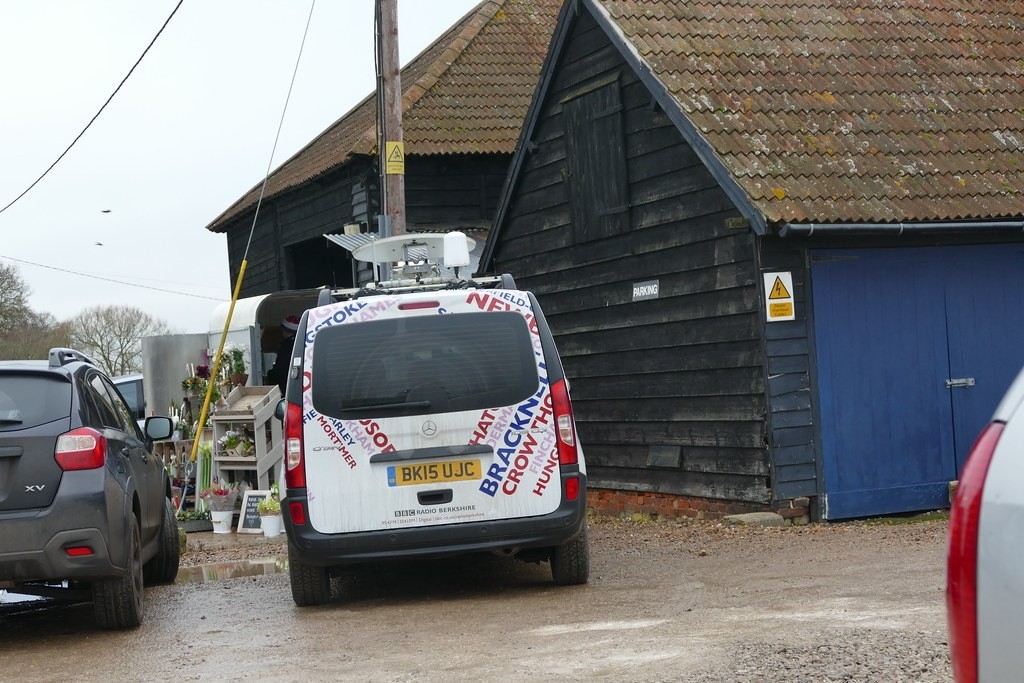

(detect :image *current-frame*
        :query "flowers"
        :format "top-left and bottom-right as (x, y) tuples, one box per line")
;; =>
(179, 347), (231, 427)
(201, 480), (239, 512)
(167, 402), (191, 431)
(168, 442), (194, 463)
(180, 482), (195, 495)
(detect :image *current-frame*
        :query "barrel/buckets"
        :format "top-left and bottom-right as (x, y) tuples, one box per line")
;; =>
(259, 515), (281, 537)
(209, 511), (233, 534)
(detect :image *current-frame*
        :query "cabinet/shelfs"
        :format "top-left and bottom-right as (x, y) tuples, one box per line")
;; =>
(212, 384), (283, 513)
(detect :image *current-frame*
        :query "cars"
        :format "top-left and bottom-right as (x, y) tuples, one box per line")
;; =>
(945, 365), (1023, 683)
(106, 373), (146, 440)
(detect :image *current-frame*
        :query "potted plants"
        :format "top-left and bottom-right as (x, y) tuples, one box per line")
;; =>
(228, 349), (249, 387)
(175, 509), (214, 533)
(227, 436), (246, 459)
(256, 498), (283, 538)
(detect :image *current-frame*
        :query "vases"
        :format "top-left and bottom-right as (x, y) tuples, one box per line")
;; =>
(211, 511), (231, 535)
(172, 430), (184, 442)
(171, 461), (197, 478)
(184, 495), (197, 504)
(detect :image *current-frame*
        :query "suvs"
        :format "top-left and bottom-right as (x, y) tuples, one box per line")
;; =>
(0, 347), (180, 629)
(280, 229), (589, 607)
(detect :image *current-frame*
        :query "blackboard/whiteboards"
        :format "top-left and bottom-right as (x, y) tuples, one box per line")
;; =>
(237, 490), (271, 534)
